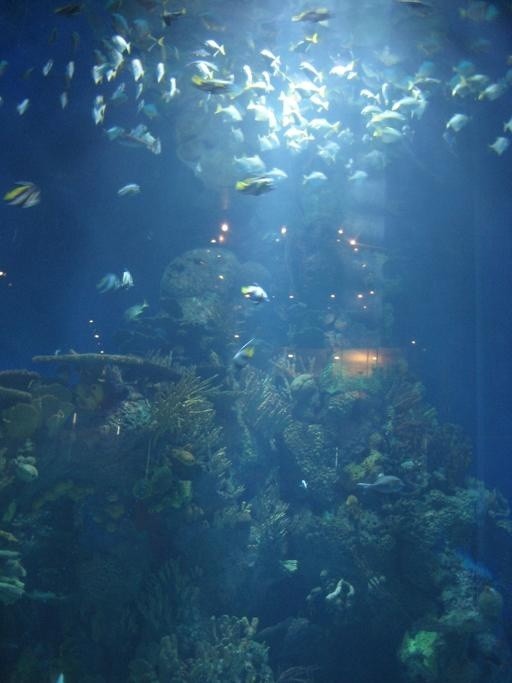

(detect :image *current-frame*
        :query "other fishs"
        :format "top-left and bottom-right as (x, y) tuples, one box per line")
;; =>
(43, 0), (512, 197)
